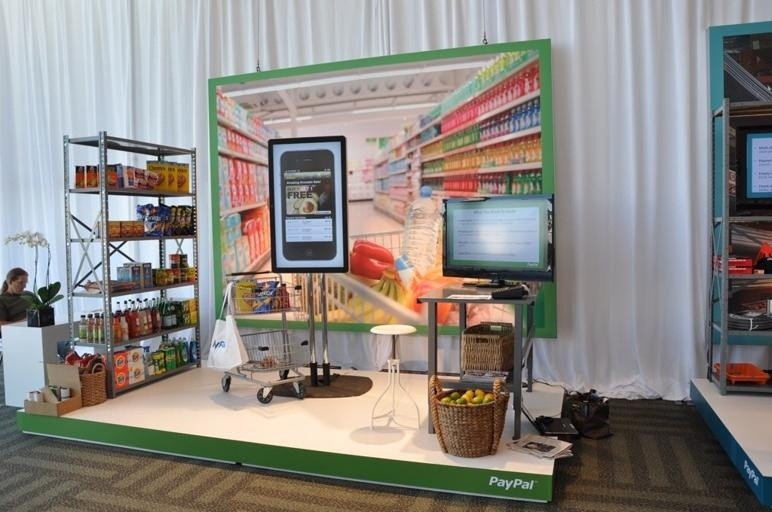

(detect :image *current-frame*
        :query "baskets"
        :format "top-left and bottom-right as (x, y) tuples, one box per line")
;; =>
(78, 363), (108, 406)
(428, 374), (509, 457)
(24, 395), (80, 416)
(461, 322), (513, 372)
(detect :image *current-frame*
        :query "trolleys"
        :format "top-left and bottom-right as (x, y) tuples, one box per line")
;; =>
(217, 270), (309, 403)
(294, 227), (436, 324)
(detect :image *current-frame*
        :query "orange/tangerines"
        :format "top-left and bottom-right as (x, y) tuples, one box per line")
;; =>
(441, 389), (493, 405)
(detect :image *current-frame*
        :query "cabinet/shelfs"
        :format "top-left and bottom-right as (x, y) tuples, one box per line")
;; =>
(707, 96), (772, 396)
(348, 55), (541, 237)
(60, 130), (201, 399)
(214, 90), (282, 290)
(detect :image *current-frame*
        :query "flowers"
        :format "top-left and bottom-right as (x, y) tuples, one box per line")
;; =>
(2, 231), (65, 308)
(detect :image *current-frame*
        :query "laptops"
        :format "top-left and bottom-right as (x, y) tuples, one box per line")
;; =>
(520, 401), (580, 436)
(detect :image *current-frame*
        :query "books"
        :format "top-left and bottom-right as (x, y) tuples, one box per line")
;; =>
(729, 223), (772, 274)
(515, 433), (575, 458)
(729, 279), (771, 332)
(506, 434), (526, 448)
(723, 50), (772, 102)
(535, 415), (579, 438)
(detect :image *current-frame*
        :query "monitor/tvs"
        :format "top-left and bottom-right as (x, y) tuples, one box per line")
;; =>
(736, 125), (772, 210)
(442, 194), (554, 288)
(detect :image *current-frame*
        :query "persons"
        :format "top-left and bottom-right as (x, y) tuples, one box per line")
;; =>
(318, 178), (332, 211)
(0, 268), (42, 339)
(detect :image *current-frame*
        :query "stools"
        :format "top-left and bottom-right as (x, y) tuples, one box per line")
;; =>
(369, 324), (423, 429)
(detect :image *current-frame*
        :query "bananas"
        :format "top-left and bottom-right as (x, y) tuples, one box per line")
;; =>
(316, 272), (407, 323)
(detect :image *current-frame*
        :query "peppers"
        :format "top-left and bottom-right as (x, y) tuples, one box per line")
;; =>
(349, 240), (397, 279)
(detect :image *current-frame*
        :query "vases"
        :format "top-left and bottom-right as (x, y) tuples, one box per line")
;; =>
(26, 308), (56, 327)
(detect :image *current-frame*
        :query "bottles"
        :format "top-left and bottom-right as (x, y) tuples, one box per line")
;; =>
(79, 296), (178, 343)
(374, 51), (540, 200)
(143, 335), (197, 375)
(402, 186), (440, 276)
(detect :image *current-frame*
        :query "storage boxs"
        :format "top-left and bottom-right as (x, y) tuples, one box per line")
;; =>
(23, 363), (83, 417)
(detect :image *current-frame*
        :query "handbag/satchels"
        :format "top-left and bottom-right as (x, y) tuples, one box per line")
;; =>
(561, 390), (613, 439)
(207, 282), (249, 370)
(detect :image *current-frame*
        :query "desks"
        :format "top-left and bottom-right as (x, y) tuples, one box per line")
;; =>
(1, 313), (82, 409)
(416, 280), (543, 440)
